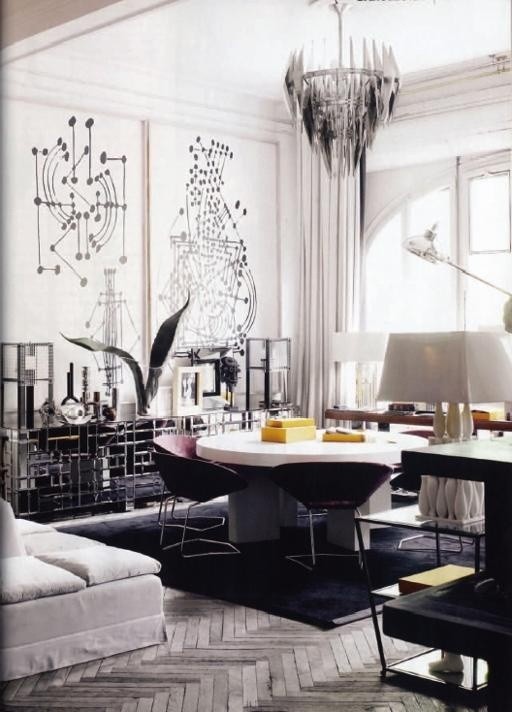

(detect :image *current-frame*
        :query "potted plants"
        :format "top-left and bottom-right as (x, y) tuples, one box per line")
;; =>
(59, 289), (191, 418)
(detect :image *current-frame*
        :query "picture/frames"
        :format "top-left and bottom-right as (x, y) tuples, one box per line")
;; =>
(171, 365), (202, 417)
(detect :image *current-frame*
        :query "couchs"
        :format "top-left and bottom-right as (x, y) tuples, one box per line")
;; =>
(1, 496), (168, 684)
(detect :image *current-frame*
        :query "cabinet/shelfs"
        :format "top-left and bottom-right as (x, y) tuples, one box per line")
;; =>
(395, 438), (511, 711)
(0, 338), (299, 522)
(355, 504), (489, 707)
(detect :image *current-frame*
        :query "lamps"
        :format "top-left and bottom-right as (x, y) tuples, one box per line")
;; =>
(402, 223), (510, 330)
(281, 1), (402, 179)
(373, 331), (512, 524)
(332, 331), (384, 408)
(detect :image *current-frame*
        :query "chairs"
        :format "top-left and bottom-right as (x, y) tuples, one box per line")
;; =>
(389, 473), (474, 554)
(264, 462), (394, 571)
(151, 434), (242, 558)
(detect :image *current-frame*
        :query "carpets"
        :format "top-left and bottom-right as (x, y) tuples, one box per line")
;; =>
(52, 500), (487, 629)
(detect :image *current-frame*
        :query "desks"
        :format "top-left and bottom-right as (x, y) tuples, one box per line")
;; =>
(325, 407), (512, 433)
